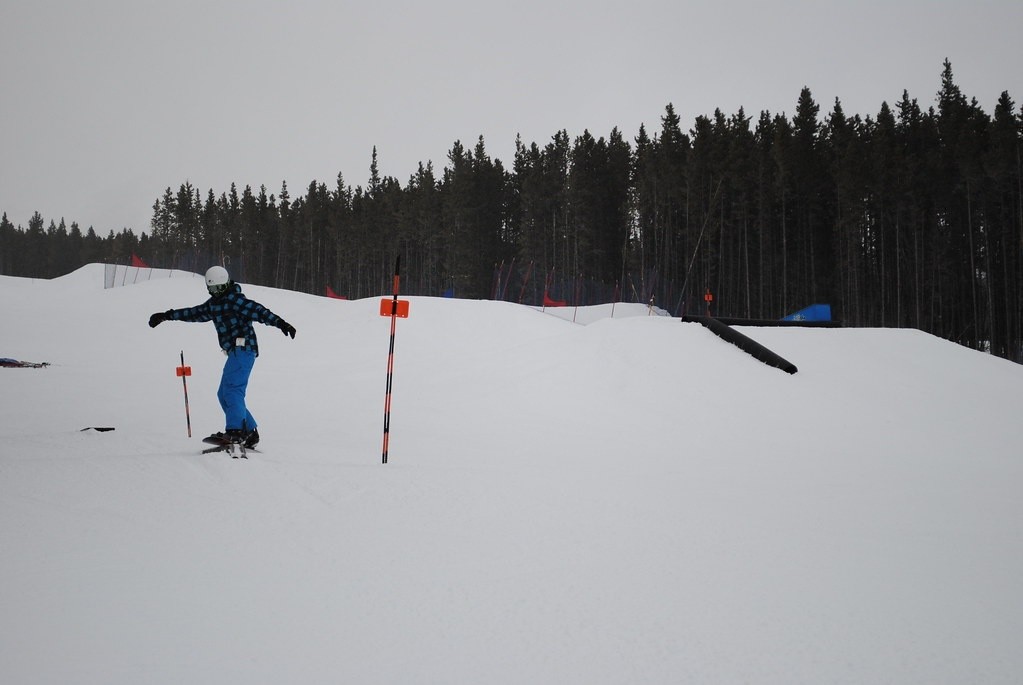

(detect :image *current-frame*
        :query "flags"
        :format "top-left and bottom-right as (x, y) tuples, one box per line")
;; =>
(442, 287), (452, 297)
(132, 254), (150, 267)
(326, 288), (347, 299)
(543, 290), (567, 306)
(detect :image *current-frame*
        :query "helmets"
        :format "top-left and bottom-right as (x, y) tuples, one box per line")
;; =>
(205, 266), (229, 286)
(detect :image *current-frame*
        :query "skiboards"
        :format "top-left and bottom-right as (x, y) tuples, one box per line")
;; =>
(202, 435), (262, 452)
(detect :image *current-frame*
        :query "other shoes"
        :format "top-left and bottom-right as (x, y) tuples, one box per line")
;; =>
(245, 429), (260, 443)
(219, 429), (243, 442)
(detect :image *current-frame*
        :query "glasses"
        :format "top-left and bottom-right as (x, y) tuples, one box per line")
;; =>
(208, 284), (227, 293)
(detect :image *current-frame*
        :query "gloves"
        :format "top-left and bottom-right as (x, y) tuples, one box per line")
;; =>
(149, 312), (166, 328)
(278, 319), (296, 339)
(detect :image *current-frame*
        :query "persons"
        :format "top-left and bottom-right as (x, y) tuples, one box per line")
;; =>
(149, 266), (297, 450)
